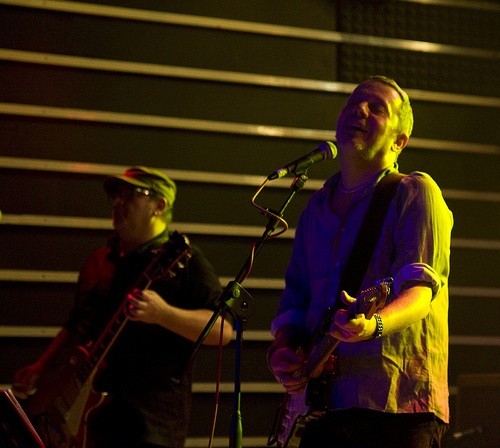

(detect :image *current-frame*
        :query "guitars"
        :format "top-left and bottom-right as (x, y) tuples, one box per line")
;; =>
(277, 281), (391, 448)
(0, 231), (192, 448)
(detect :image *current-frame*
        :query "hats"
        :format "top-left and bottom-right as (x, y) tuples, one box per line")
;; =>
(102, 165), (177, 209)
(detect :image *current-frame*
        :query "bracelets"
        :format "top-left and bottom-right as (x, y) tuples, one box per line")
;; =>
(375, 311), (383, 339)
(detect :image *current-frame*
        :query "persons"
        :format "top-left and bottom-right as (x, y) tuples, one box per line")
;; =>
(265, 75), (455, 448)
(13, 165), (235, 448)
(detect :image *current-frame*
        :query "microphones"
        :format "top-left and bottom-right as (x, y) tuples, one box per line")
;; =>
(268, 141), (338, 181)
